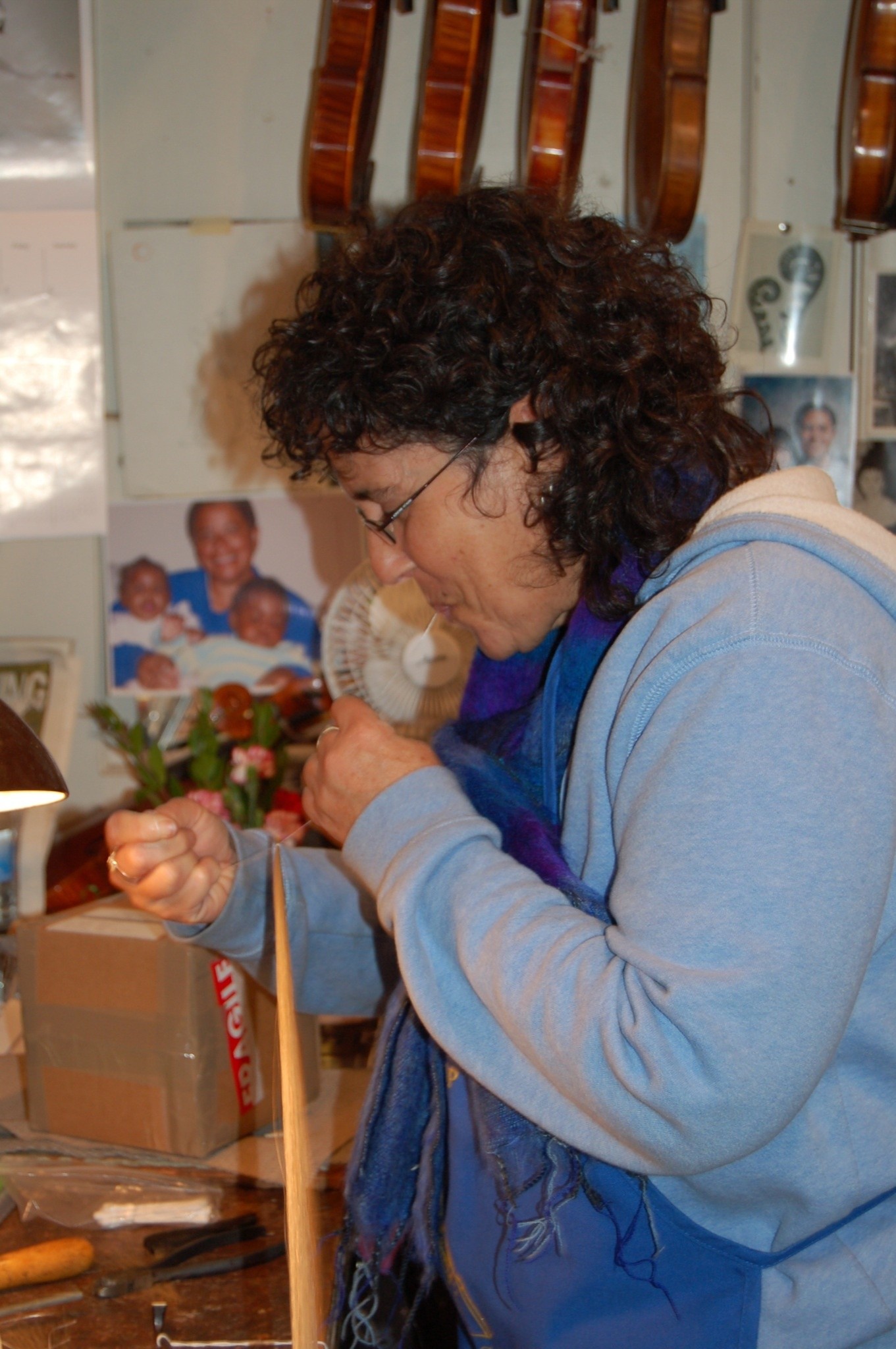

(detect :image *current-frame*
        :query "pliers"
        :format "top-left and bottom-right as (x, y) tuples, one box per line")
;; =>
(94, 1222), (287, 1298)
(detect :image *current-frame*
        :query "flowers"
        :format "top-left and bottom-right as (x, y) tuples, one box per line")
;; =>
(78, 674), (329, 830)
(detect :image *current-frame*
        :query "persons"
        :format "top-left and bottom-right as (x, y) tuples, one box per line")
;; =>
(111, 500), (321, 706)
(857, 443), (896, 528)
(763, 401), (847, 492)
(106, 185), (896, 1349)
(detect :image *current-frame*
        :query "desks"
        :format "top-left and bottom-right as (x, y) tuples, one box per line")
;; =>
(0, 1017), (378, 1349)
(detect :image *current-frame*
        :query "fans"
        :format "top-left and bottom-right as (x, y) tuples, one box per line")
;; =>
(320, 558), (474, 739)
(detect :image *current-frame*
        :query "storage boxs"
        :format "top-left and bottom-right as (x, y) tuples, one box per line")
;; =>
(13, 893), (318, 1157)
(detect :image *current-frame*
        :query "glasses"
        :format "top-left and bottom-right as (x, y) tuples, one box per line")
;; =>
(360, 438), (476, 545)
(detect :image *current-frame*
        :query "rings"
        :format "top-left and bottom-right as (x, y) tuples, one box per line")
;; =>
(316, 724), (340, 751)
(108, 852), (137, 884)
